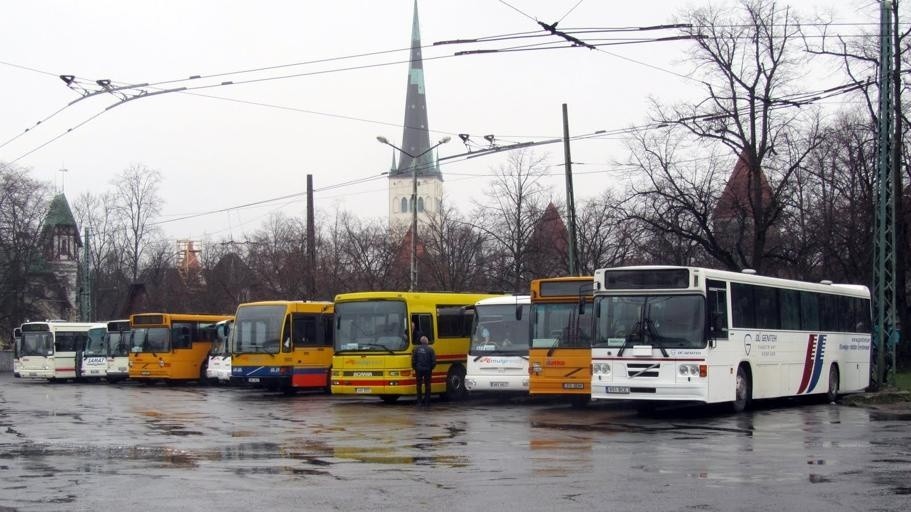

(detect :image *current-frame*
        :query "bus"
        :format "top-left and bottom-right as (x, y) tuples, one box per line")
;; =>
(18, 320), (108, 384)
(460, 294), (535, 401)
(223, 300), (335, 397)
(578, 264), (874, 416)
(119, 313), (235, 388)
(515, 276), (595, 408)
(320, 292), (510, 404)
(13, 328), (22, 378)
(80, 320), (146, 385)
(206, 318), (265, 388)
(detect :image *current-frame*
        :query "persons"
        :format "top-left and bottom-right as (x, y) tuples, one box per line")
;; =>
(412, 334), (438, 407)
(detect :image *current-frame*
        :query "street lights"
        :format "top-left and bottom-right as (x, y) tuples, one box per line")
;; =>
(377, 136), (451, 293)
